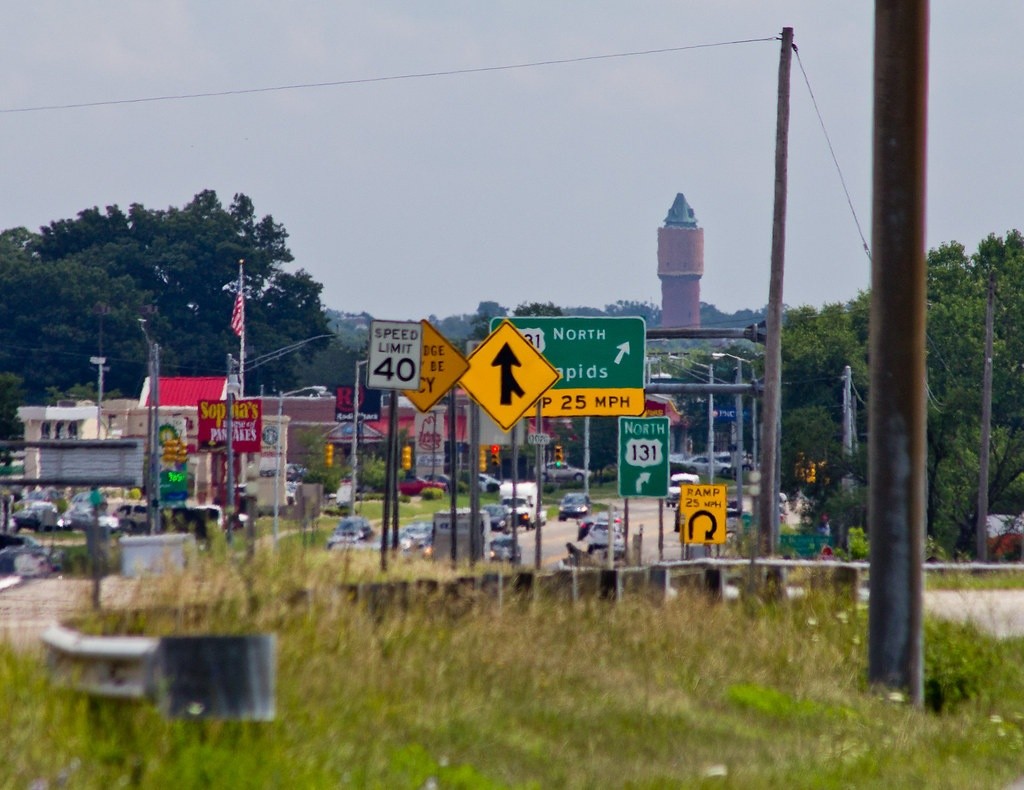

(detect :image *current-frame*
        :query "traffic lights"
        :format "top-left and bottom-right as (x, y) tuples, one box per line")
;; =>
(556, 445), (563, 466)
(402, 446), (413, 471)
(490, 444), (500, 467)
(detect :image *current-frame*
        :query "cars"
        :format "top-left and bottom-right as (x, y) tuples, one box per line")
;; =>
(328, 516), (519, 565)
(9, 496), (253, 534)
(684, 454), (755, 479)
(585, 507), (625, 554)
(381, 458), (592, 531)
(668, 468), (738, 534)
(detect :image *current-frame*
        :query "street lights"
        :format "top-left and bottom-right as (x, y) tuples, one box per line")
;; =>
(273, 386), (330, 538)
(226, 331), (340, 517)
(644, 349), (756, 509)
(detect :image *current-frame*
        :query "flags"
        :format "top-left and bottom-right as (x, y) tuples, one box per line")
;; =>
(230, 264), (246, 338)
